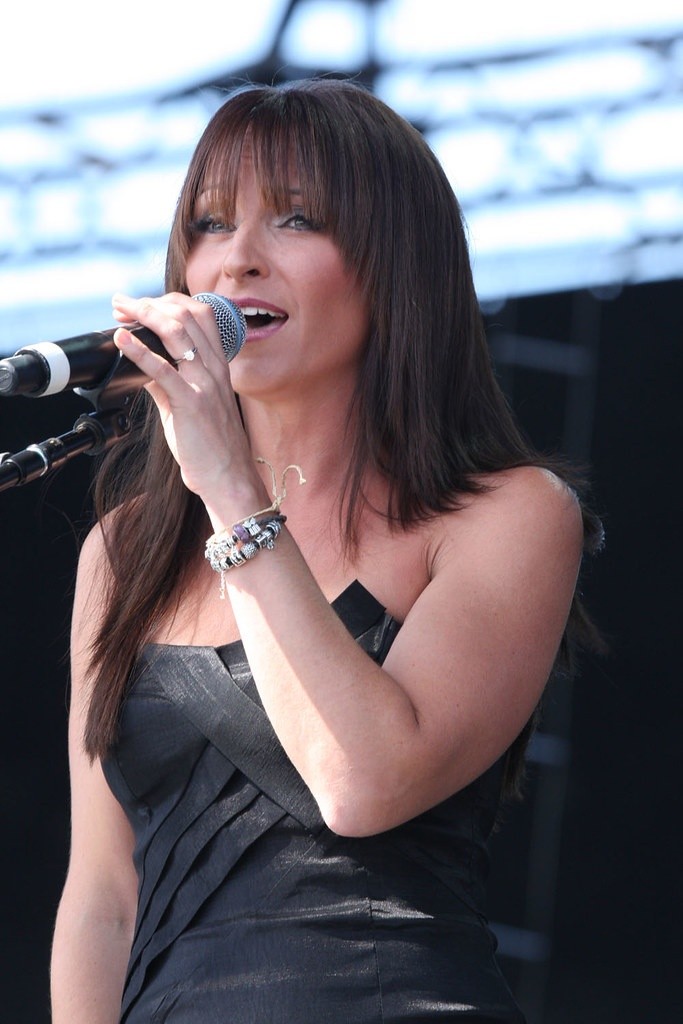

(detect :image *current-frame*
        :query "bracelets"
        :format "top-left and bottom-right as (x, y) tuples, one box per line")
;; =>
(204, 458), (306, 600)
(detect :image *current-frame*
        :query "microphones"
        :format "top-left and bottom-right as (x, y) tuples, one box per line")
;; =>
(1, 291), (246, 400)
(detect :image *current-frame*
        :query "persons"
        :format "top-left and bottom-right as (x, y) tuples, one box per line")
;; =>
(51, 82), (594, 1024)
